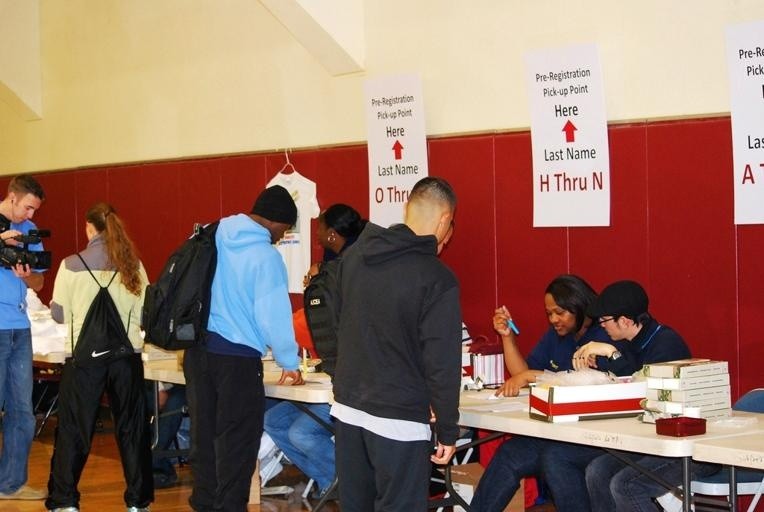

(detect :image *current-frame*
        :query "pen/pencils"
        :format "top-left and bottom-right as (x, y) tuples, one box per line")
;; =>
(303, 347), (308, 379)
(506, 320), (520, 335)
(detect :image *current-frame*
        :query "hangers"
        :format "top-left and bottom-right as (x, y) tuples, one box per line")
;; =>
(278, 145), (298, 175)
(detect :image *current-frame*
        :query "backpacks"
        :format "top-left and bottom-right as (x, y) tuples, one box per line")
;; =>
(140, 220), (218, 349)
(303, 261), (352, 379)
(72, 286), (133, 373)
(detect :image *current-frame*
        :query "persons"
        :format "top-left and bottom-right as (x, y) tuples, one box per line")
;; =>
(301, 172), (463, 511)
(312, 204), (368, 262)
(462, 271), (629, 512)
(180, 182), (307, 510)
(570, 278), (723, 511)
(0, 170), (50, 503)
(142, 377), (188, 491)
(262, 257), (338, 505)
(435, 222), (454, 257)
(44, 200), (157, 511)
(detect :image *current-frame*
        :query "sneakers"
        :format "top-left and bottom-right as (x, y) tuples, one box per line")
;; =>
(0, 485), (48, 502)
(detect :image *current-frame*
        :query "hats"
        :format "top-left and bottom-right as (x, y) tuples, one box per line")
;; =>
(250, 184), (298, 227)
(586, 280), (650, 320)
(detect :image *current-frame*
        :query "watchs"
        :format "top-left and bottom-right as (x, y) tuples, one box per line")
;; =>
(607, 350), (622, 361)
